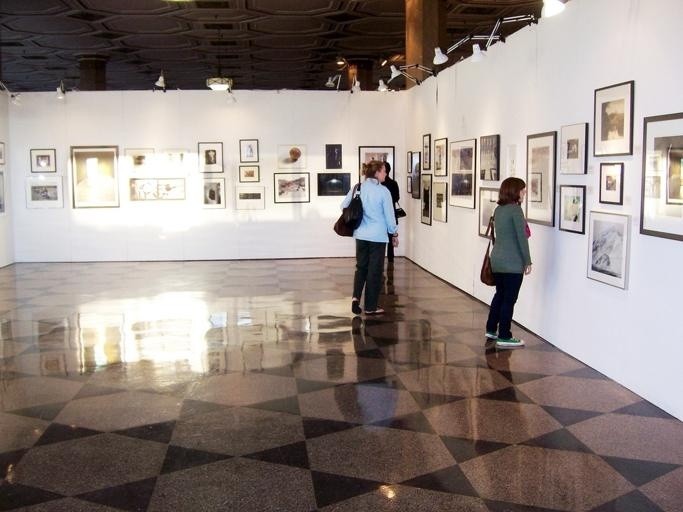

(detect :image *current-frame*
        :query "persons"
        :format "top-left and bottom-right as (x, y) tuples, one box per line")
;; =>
(379, 160), (400, 274)
(340, 159), (399, 315)
(606, 176), (614, 189)
(485, 176), (533, 347)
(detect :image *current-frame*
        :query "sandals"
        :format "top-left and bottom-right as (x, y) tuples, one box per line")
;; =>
(351, 298), (362, 314)
(363, 306), (385, 315)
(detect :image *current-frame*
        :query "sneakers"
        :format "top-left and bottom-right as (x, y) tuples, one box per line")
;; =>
(483, 330), (498, 340)
(495, 335), (525, 347)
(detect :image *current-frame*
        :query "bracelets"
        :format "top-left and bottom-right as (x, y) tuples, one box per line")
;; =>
(393, 233), (398, 237)
(525, 263), (532, 266)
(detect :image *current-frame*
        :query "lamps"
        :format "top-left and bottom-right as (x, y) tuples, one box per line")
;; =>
(150, 66), (169, 93)
(205, 58), (232, 93)
(56, 80), (81, 100)
(325, 0), (566, 99)
(0, 81), (21, 107)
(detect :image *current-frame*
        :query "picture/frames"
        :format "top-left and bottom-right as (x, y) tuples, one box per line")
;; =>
(0, 169), (6, 217)
(407, 150), (420, 200)
(431, 182), (448, 223)
(556, 121), (591, 179)
(557, 183), (588, 234)
(196, 141), (226, 174)
(67, 144), (121, 209)
(324, 143), (344, 170)
(356, 144), (398, 186)
(235, 183), (266, 208)
(316, 172), (352, 197)
(238, 137), (260, 163)
(591, 81), (637, 159)
(421, 132), (432, 171)
(522, 129), (557, 230)
(238, 164), (260, 183)
(273, 143), (308, 171)
(29, 175), (64, 209)
(128, 177), (187, 202)
(123, 148), (156, 169)
(596, 161), (627, 207)
(420, 173), (431, 227)
(432, 136), (448, 178)
(448, 137), (477, 211)
(30, 148), (58, 173)
(478, 186), (528, 243)
(637, 110), (683, 244)
(203, 177), (228, 208)
(585, 209), (635, 292)
(272, 171), (311, 205)
(478, 133), (501, 182)
(0, 142), (5, 165)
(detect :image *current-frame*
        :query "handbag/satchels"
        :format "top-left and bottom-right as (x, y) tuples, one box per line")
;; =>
(393, 201), (406, 218)
(480, 253), (496, 287)
(333, 182), (365, 238)
